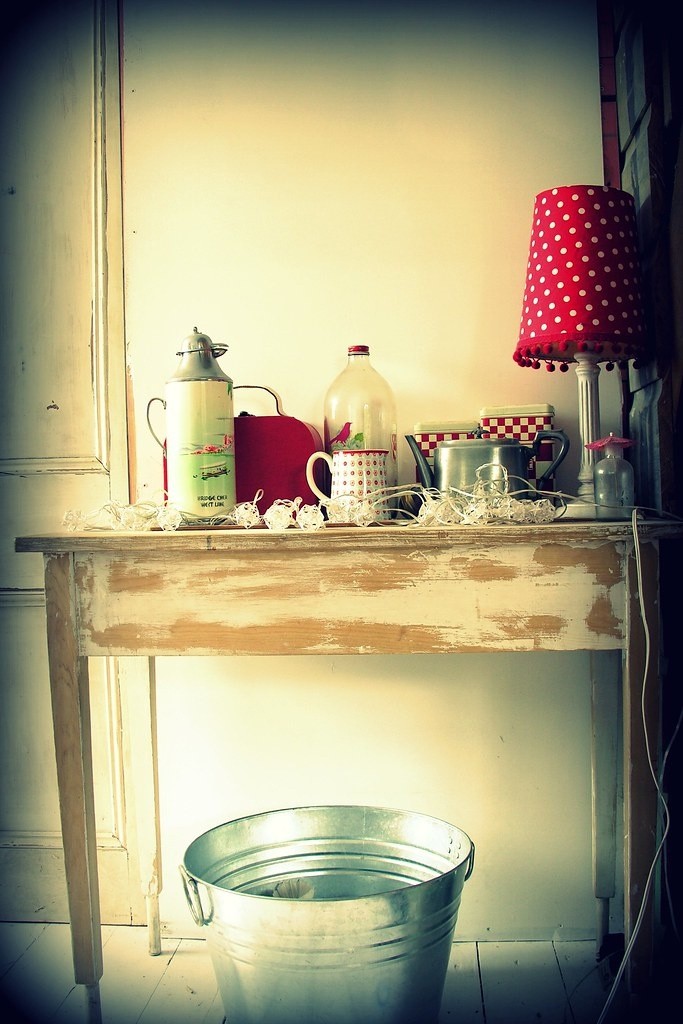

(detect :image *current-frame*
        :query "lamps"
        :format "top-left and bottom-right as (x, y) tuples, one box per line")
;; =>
(512, 185), (649, 520)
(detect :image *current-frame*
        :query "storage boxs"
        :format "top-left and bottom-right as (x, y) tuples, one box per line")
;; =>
(479, 403), (558, 508)
(414, 420), (479, 490)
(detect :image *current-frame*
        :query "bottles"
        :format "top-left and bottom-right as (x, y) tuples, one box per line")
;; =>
(324, 345), (399, 519)
(593, 444), (635, 520)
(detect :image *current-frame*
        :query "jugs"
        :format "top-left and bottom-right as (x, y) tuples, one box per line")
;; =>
(146, 327), (237, 524)
(405, 429), (571, 506)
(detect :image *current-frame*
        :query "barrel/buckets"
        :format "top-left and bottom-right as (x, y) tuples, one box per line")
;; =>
(180, 805), (476, 1024)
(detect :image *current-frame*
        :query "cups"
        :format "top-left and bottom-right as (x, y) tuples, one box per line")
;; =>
(305, 449), (392, 522)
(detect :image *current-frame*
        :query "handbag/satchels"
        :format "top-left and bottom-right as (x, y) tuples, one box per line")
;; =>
(162, 385), (324, 521)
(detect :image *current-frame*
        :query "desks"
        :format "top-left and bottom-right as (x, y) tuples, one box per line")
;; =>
(13, 518), (682, 1024)
